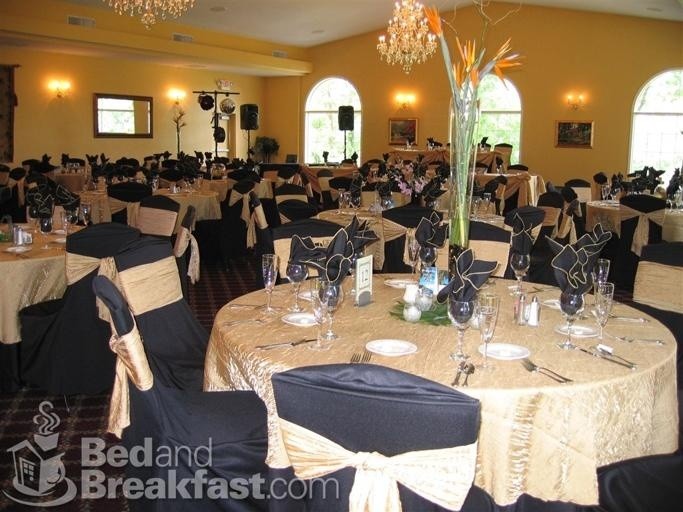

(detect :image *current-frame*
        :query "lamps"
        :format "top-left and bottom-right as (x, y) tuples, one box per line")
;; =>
(101, 1), (200, 32)
(376, 1), (438, 76)
(566, 95), (584, 111)
(195, 90), (241, 157)
(396, 94), (414, 111)
(48, 81), (70, 99)
(170, 90), (185, 105)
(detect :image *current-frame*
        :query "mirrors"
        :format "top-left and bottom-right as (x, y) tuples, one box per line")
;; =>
(92, 93), (154, 139)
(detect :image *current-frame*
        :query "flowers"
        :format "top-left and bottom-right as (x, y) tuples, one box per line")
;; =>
(424, 5), (521, 212)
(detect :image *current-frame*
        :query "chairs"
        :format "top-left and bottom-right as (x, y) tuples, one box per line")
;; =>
(0, 141), (682, 512)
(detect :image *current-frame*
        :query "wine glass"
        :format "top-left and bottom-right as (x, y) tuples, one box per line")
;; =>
(60, 160), (228, 192)
(219, 236), (668, 392)
(337, 190), (395, 219)
(354, 162), (393, 187)
(430, 191), (497, 223)
(404, 139), (491, 153)
(1, 203), (89, 253)
(600, 173), (683, 214)
(450, 158), (507, 175)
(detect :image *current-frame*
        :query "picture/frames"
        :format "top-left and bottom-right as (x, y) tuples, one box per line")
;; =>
(553, 120), (594, 150)
(388, 117), (419, 145)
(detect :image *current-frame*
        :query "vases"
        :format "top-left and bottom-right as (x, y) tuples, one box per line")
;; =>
(447, 97), (479, 250)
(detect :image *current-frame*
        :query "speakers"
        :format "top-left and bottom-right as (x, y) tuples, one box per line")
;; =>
(239, 103), (258, 131)
(339, 106), (354, 131)
